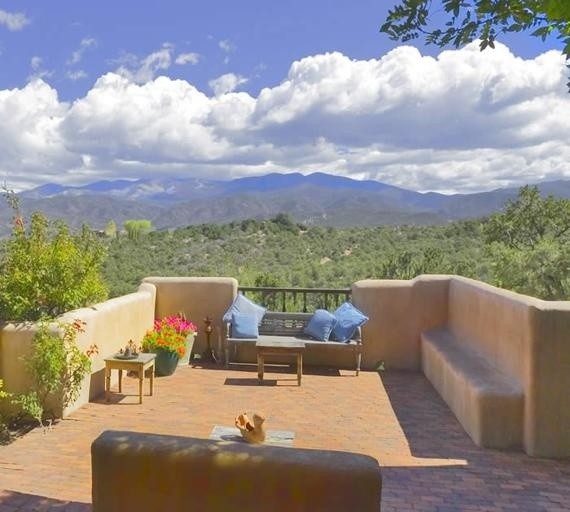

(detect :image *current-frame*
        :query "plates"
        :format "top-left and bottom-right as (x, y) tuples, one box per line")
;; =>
(115, 351), (140, 359)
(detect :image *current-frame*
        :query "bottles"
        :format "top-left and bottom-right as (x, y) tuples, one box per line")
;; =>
(124, 348), (131, 357)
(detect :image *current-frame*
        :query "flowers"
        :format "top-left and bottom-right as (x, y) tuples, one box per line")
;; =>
(153, 312), (198, 334)
(140, 327), (188, 358)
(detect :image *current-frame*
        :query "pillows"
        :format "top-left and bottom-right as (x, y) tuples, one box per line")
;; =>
(232, 313), (260, 339)
(302, 307), (338, 342)
(222, 294), (268, 322)
(327, 301), (371, 345)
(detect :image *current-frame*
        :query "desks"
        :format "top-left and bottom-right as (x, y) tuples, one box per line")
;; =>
(103, 352), (159, 403)
(208, 421), (298, 449)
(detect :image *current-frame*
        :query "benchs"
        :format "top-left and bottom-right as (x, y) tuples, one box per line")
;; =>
(223, 310), (363, 378)
(91, 430), (384, 512)
(419, 328), (525, 453)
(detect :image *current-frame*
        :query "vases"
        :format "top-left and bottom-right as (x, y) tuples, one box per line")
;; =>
(144, 347), (179, 377)
(175, 331), (198, 367)
